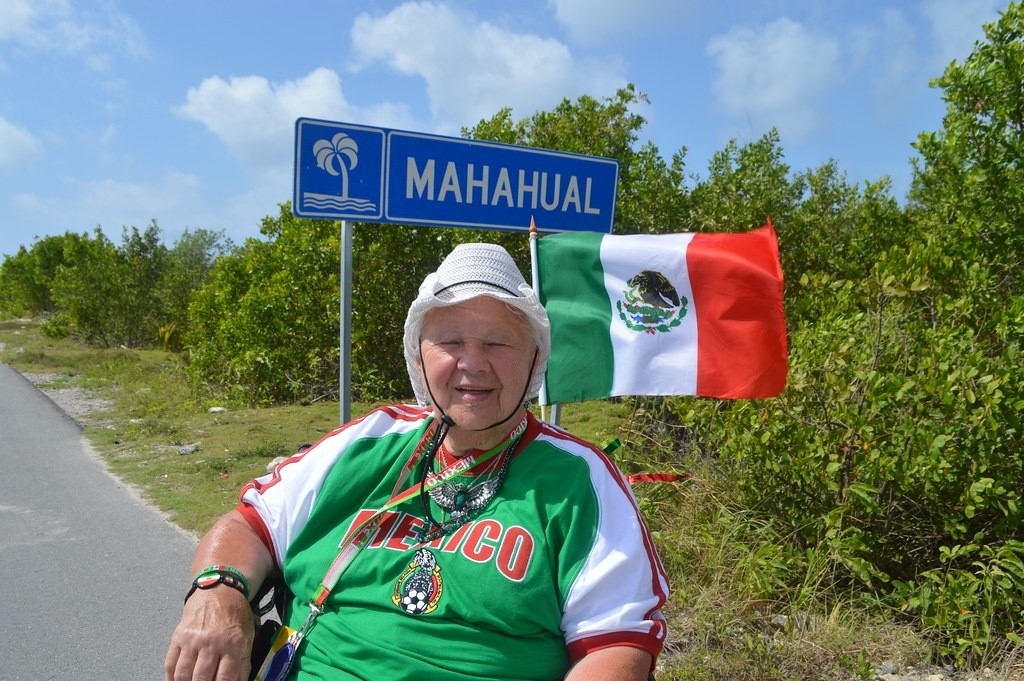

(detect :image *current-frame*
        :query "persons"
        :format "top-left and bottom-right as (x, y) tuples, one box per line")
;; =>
(167, 243), (670, 681)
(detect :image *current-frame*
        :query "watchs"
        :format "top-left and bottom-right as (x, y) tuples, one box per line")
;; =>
(185, 571), (249, 605)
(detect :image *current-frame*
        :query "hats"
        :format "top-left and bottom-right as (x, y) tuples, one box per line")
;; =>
(401, 242), (550, 409)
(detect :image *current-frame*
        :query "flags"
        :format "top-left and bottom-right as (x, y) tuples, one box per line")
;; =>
(539, 216), (787, 406)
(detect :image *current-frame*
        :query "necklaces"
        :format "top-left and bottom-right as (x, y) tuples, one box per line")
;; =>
(417, 422), (533, 545)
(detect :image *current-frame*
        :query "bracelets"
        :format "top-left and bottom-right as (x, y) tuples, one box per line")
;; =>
(193, 565), (250, 591)
